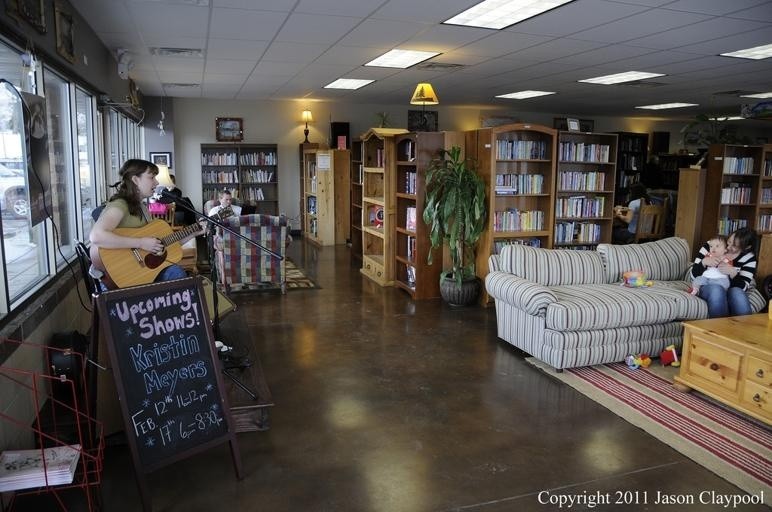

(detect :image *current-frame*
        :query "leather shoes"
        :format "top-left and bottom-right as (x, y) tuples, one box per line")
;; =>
(218, 346), (248, 360)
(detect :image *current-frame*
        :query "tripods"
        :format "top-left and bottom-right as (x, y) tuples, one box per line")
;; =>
(208, 226), (257, 400)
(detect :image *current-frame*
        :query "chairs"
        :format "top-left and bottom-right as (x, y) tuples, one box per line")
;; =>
(74, 243), (104, 305)
(214, 214), (289, 296)
(206, 197), (237, 216)
(148, 202), (177, 233)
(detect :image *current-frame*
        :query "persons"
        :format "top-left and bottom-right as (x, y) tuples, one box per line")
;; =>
(88, 159), (233, 356)
(689, 228), (758, 318)
(614, 182), (654, 242)
(691, 234), (733, 295)
(208, 190), (241, 217)
(160, 175), (182, 197)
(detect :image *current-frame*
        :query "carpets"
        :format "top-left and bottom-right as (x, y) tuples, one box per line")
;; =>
(522, 349), (772, 511)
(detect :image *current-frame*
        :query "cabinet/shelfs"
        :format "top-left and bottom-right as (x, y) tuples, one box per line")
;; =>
(299, 117), (771, 312)
(198, 144), (280, 217)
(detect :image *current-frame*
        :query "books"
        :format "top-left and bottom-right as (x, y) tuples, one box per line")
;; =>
(306, 160), (317, 238)
(718, 156), (772, 237)
(405, 141), (418, 289)
(202, 150), (276, 202)
(496, 138), (645, 250)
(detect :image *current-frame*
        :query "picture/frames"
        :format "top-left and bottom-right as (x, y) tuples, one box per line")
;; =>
(149, 151), (173, 169)
(52, 5), (77, 63)
(12, 0), (46, 32)
(215, 116), (244, 142)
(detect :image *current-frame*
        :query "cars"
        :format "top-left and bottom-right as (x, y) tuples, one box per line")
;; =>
(81, 183), (92, 209)
(1, 161), (30, 219)
(1, 157), (25, 175)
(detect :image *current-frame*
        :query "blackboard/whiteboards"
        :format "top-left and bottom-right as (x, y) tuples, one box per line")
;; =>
(97, 273), (236, 476)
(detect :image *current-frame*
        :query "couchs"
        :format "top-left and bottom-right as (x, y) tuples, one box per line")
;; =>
(484, 232), (767, 380)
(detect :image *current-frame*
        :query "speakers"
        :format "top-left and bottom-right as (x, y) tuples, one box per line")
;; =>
(331, 122), (350, 148)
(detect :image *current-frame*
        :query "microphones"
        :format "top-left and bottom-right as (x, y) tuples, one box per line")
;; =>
(155, 186), (178, 199)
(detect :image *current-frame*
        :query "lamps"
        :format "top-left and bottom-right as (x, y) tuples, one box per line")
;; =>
(300, 111), (314, 143)
(410, 81), (440, 132)
(156, 89), (169, 138)
(151, 165), (176, 189)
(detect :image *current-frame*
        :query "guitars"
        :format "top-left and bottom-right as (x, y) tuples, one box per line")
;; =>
(90, 204), (234, 290)
